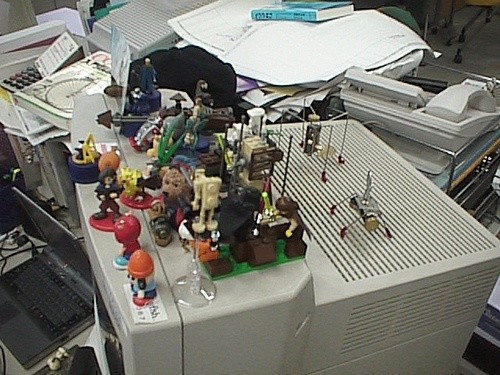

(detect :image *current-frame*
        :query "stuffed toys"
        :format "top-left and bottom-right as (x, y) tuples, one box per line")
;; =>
(159, 166), (194, 201)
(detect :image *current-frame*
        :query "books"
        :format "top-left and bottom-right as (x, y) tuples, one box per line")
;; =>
(12, 50), (113, 129)
(250, 0), (356, 25)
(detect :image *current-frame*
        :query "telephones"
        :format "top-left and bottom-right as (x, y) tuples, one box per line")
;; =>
(339, 67), (500, 153)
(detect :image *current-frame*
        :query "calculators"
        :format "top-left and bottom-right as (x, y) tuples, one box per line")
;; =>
(0, 65), (42, 92)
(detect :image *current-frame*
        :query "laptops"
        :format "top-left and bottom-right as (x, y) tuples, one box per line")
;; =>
(0, 186), (96, 371)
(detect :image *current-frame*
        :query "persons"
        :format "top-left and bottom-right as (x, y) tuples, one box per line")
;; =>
(113, 214), (144, 272)
(93, 167), (124, 223)
(126, 249), (157, 307)
(139, 58), (157, 94)
(273, 195), (311, 242)
(218, 187), (262, 241)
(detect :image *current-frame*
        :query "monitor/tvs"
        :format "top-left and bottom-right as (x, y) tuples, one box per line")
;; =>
(70, 93), (500, 375)
